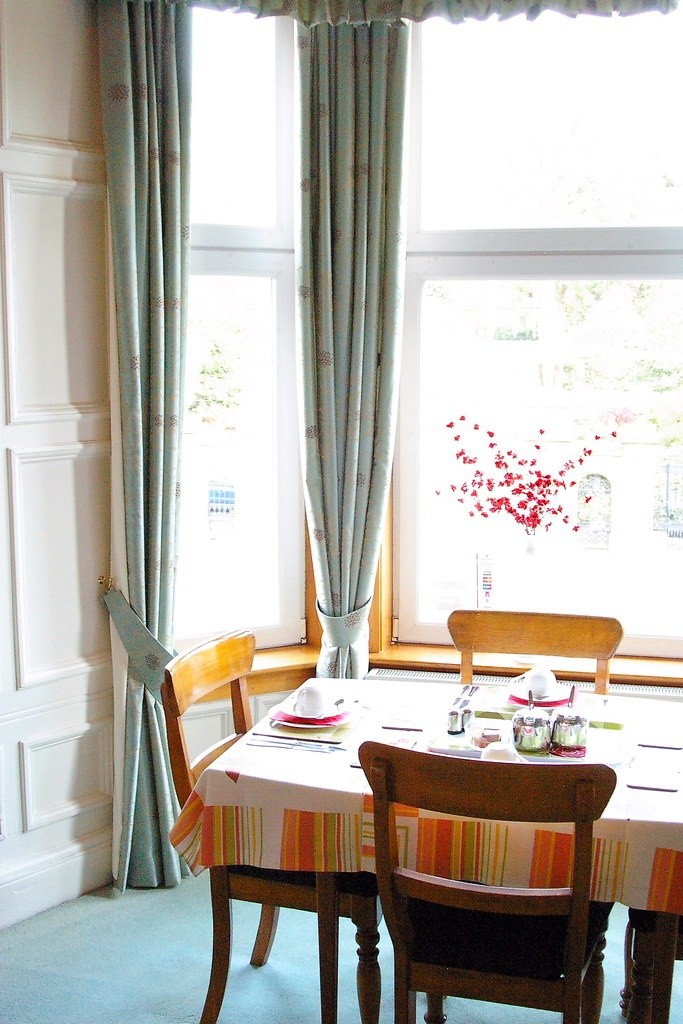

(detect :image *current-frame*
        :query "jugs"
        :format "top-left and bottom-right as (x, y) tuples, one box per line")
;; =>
(511, 689), (551, 751)
(549, 685), (589, 757)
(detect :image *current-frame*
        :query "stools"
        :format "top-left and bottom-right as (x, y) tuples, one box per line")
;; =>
(618, 904), (683, 1024)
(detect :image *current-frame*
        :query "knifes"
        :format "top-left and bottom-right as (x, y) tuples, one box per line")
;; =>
(452, 685), (479, 708)
(246, 737), (347, 753)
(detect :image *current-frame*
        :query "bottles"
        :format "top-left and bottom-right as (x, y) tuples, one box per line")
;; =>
(447, 708), (473, 735)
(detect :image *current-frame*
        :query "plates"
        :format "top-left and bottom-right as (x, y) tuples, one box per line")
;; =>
(268, 701), (352, 729)
(508, 683), (574, 703)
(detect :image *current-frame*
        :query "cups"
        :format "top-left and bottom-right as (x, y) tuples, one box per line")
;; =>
(293, 684), (326, 717)
(480, 742), (523, 764)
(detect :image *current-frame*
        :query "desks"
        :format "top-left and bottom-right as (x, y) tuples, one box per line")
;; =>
(169, 679), (682, 1024)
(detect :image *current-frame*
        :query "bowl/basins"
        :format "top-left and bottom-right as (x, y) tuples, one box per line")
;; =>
(522, 666), (558, 697)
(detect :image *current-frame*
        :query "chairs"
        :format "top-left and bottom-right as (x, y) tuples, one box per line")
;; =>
(447, 609), (624, 695)
(161, 630), (382, 1024)
(359, 742), (614, 1024)
